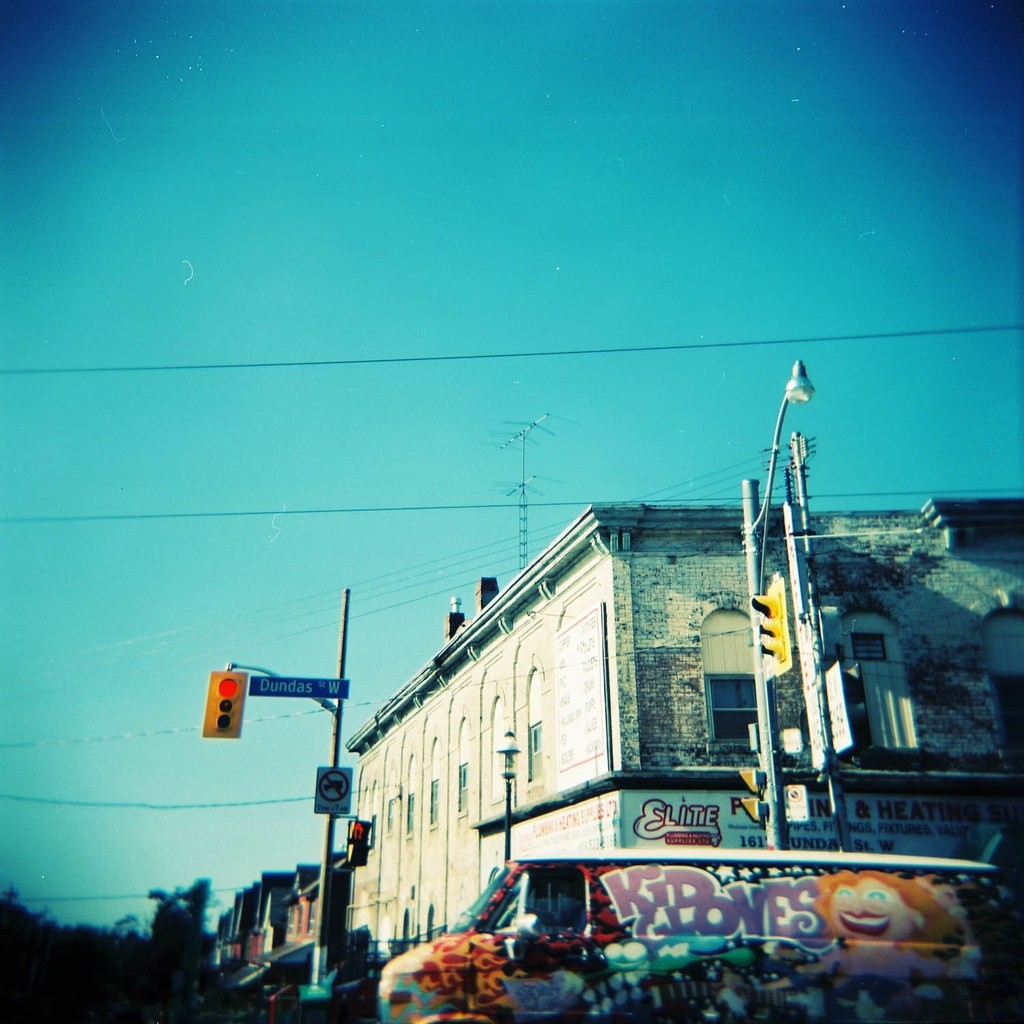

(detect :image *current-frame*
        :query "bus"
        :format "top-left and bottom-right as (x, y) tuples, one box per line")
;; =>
(376, 846), (1024, 1024)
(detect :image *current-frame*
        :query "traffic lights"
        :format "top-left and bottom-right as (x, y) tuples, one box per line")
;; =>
(753, 579), (793, 681)
(202, 671), (249, 739)
(738, 768), (762, 825)
(345, 819), (373, 868)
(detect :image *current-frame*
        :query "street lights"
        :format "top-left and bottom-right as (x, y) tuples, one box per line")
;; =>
(497, 731), (523, 862)
(743, 358), (816, 849)
